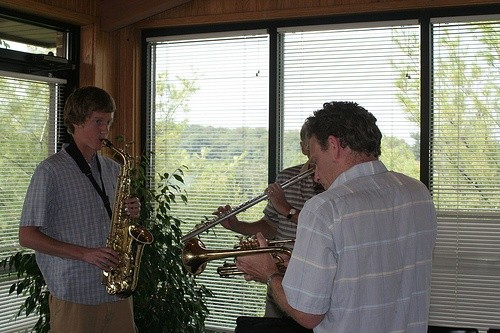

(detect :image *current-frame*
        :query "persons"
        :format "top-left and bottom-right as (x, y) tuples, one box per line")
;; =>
(18, 86), (141, 333)
(235, 100), (437, 333)
(212, 118), (326, 317)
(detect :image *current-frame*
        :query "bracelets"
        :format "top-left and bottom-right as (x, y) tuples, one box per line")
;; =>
(266, 273), (283, 290)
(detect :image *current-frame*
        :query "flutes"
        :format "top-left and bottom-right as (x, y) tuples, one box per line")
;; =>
(180, 166), (316, 244)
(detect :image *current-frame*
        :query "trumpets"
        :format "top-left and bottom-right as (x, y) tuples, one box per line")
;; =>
(182, 238), (296, 275)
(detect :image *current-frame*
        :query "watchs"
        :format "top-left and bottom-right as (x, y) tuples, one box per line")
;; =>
(286, 207), (297, 220)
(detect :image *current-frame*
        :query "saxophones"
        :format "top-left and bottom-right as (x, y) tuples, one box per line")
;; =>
(99, 138), (154, 298)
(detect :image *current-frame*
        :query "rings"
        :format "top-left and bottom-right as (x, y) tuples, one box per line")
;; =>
(270, 190), (274, 192)
